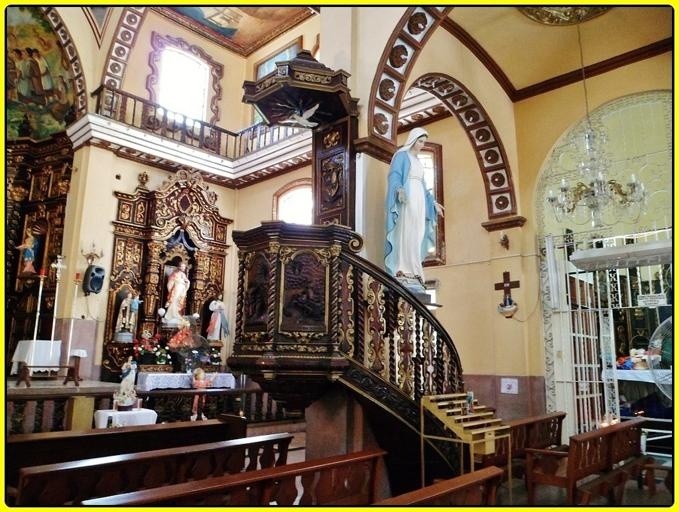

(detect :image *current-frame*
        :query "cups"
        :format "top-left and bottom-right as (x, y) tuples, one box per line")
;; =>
(461, 403), (471, 414)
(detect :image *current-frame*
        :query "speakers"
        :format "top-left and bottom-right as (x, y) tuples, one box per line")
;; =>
(82, 264), (106, 294)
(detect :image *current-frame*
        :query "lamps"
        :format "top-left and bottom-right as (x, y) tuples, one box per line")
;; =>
(543, 9), (648, 230)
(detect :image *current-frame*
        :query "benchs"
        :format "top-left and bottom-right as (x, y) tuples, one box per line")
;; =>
(482, 410), (656, 504)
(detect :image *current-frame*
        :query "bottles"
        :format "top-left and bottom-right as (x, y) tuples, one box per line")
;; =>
(466, 384), (474, 413)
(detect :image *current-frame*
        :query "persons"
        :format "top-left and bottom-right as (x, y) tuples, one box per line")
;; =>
(188, 367), (219, 421)
(23, 227), (40, 272)
(165, 262), (192, 321)
(207, 291), (226, 341)
(117, 290), (138, 332)
(129, 294), (144, 325)
(115, 354), (138, 406)
(9, 28), (77, 137)
(383, 127), (445, 280)
